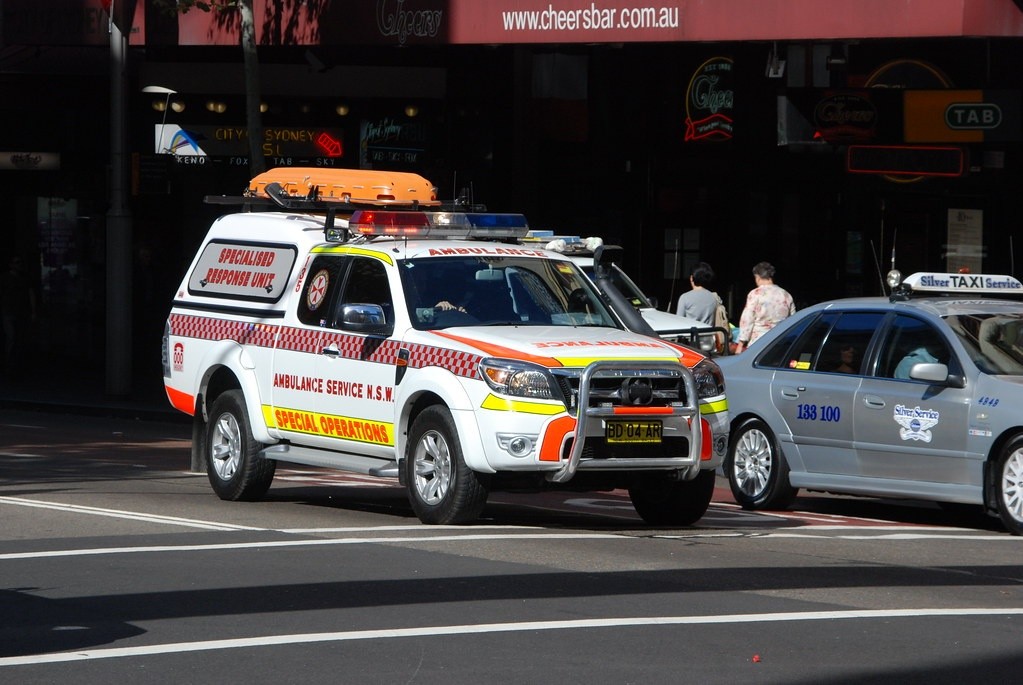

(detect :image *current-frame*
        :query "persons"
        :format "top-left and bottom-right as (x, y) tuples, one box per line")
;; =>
(735, 262), (798, 356)
(0, 253), (38, 385)
(383, 269), (468, 324)
(677, 266), (719, 355)
(892, 314), (1021, 380)
(826, 334), (865, 375)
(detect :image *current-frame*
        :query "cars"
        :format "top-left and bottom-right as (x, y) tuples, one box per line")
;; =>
(711, 230), (1023, 536)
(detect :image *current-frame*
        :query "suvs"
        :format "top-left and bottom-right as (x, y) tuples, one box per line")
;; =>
(515, 230), (729, 374)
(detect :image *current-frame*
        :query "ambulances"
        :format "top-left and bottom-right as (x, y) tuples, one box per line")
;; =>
(162, 167), (730, 527)
